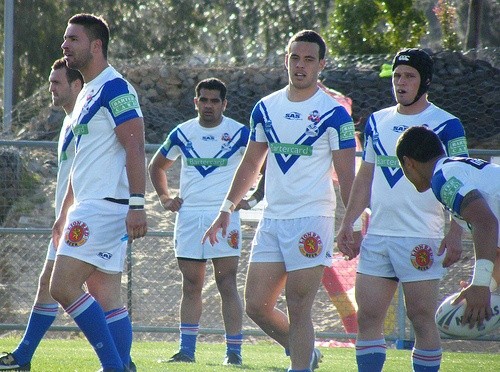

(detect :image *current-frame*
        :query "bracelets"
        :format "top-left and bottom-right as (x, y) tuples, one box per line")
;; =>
(220, 199), (236, 214)
(247, 196), (257, 208)
(352, 217), (362, 232)
(489, 277), (497, 292)
(129, 193), (145, 210)
(472, 259), (494, 286)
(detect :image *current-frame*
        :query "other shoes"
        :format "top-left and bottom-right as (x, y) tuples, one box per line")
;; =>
(102, 363), (130, 372)
(308, 348), (322, 372)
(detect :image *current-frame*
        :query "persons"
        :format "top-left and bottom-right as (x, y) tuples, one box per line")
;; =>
(50, 13), (147, 372)
(336, 48), (469, 372)
(201, 30), (363, 372)
(0, 57), (137, 372)
(396, 125), (500, 329)
(148, 78), (266, 367)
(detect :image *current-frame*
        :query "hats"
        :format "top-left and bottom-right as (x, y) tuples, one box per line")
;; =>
(393, 48), (432, 110)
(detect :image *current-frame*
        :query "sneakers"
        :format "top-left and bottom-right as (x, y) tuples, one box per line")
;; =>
(159, 353), (195, 363)
(0, 352), (31, 372)
(223, 353), (242, 366)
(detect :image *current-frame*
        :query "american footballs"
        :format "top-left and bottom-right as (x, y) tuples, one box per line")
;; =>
(434, 292), (500, 339)
